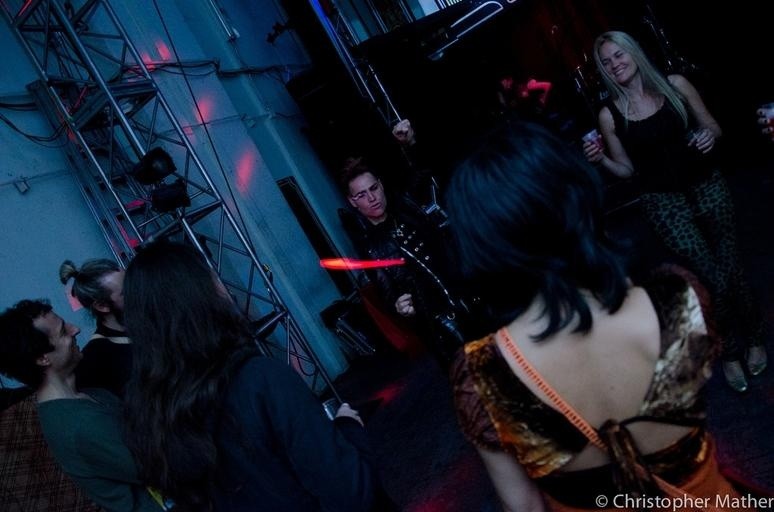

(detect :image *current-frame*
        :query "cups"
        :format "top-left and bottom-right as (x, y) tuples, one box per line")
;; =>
(321, 397), (341, 422)
(582, 129), (605, 154)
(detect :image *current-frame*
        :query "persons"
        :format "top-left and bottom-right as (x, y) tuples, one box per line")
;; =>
(120, 242), (402, 512)
(445, 123), (757, 512)
(60, 258), (132, 401)
(582, 31), (767, 391)
(0, 299), (179, 512)
(756, 108), (774, 135)
(339, 119), (494, 377)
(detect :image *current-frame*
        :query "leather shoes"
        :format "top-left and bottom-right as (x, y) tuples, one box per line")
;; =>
(747, 350), (768, 375)
(722, 360), (748, 392)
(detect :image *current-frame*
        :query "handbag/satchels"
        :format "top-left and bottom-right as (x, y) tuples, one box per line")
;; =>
(652, 430), (758, 512)
